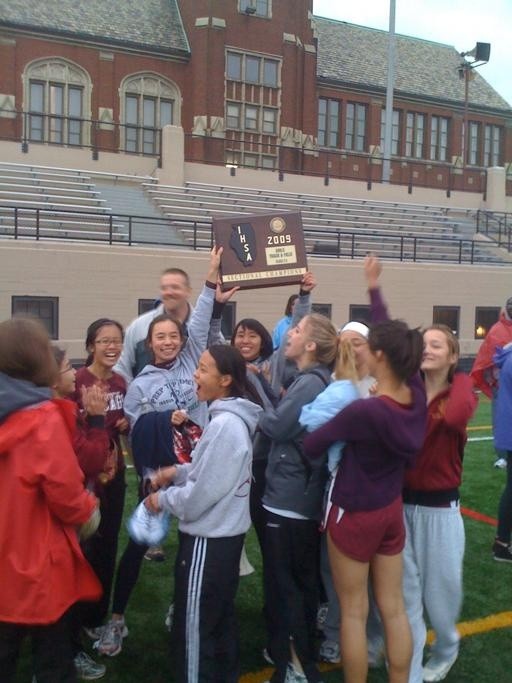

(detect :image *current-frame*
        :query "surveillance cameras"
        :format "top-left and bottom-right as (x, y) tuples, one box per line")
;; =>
(296, 37), (302, 49)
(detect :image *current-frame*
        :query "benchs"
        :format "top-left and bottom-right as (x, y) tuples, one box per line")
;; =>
(0, 159), (512, 265)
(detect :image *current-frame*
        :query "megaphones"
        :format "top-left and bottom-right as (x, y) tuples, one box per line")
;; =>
(461, 41), (491, 62)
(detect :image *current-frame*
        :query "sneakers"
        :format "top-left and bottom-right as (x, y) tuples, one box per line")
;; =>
(491, 536), (512, 563)
(315, 607), (331, 631)
(262, 647), (310, 683)
(319, 638), (343, 664)
(166, 601), (176, 632)
(422, 649), (458, 683)
(143, 546), (168, 562)
(494, 458), (508, 469)
(70, 653), (107, 681)
(81, 617), (132, 658)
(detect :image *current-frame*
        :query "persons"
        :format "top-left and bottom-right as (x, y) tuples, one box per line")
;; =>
(0, 244), (512, 682)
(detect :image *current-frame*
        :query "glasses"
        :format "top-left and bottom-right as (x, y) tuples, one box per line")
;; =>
(95, 337), (123, 346)
(59, 362), (72, 376)
